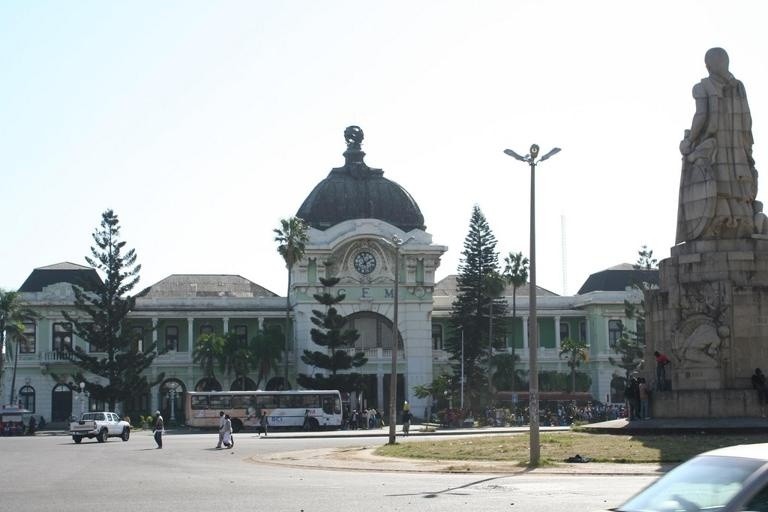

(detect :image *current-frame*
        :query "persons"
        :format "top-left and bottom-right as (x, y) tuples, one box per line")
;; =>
(341, 406), (384, 430)
(152, 410), (164, 450)
(753, 368), (768, 400)
(220, 416), (234, 447)
(679, 47), (758, 235)
(258, 411), (268, 438)
(3, 416), (45, 436)
(215, 411), (226, 449)
(438, 377), (650, 427)
(654, 351), (672, 385)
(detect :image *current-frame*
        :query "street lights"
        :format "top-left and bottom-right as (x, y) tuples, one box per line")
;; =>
(80, 381), (86, 413)
(166, 388), (180, 422)
(382, 233), (416, 441)
(456, 325), (464, 409)
(504, 144), (563, 463)
(442, 389), (458, 411)
(25, 375), (31, 409)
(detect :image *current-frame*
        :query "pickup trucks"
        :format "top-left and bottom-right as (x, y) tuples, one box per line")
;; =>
(67, 412), (132, 443)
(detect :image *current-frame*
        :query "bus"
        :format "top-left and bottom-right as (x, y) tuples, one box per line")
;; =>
(183, 389), (344, 431)
(497, 391), (594, 418)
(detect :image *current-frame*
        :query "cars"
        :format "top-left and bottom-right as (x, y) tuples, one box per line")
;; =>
(606, 443), (768, 511)
